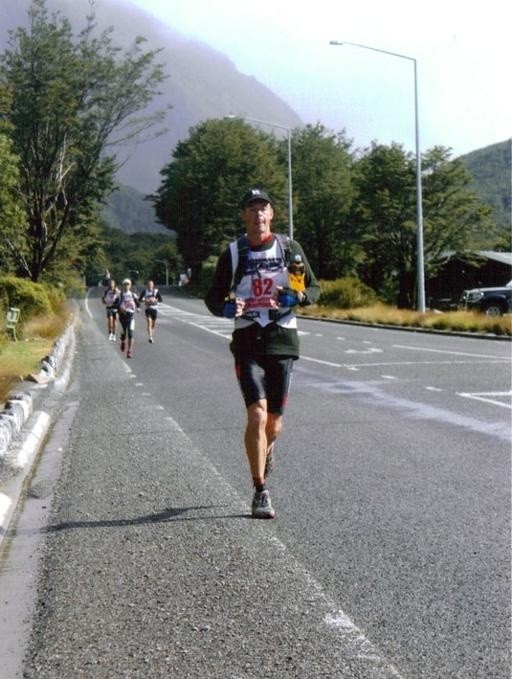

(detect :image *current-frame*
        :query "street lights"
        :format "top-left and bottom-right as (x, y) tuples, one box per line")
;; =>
(329, 39), (427, 320)
(155, 259), (169, 286)
(223, 114), (294, 240)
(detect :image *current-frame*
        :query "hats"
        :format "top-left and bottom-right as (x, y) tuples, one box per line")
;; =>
(240, 189), (272, 206)
(122, 279), (132, 285)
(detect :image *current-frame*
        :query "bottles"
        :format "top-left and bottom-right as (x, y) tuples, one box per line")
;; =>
(288, 254), (306, 292)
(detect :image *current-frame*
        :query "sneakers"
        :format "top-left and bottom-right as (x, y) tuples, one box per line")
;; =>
(264, 455), (274, 479)
(148, 338), (153, 343)
(127, 351), (133, 358)
(252, 490), (275, 519)
(120, 342), (126, 351)
(108, 333), (116, 341)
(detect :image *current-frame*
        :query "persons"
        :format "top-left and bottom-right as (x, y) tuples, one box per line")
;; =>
(102, 269), (162, 359)
(205, 189), (321, 517)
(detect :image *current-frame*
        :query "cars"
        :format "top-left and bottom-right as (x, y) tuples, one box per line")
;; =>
(462, 280), (512, 320)
